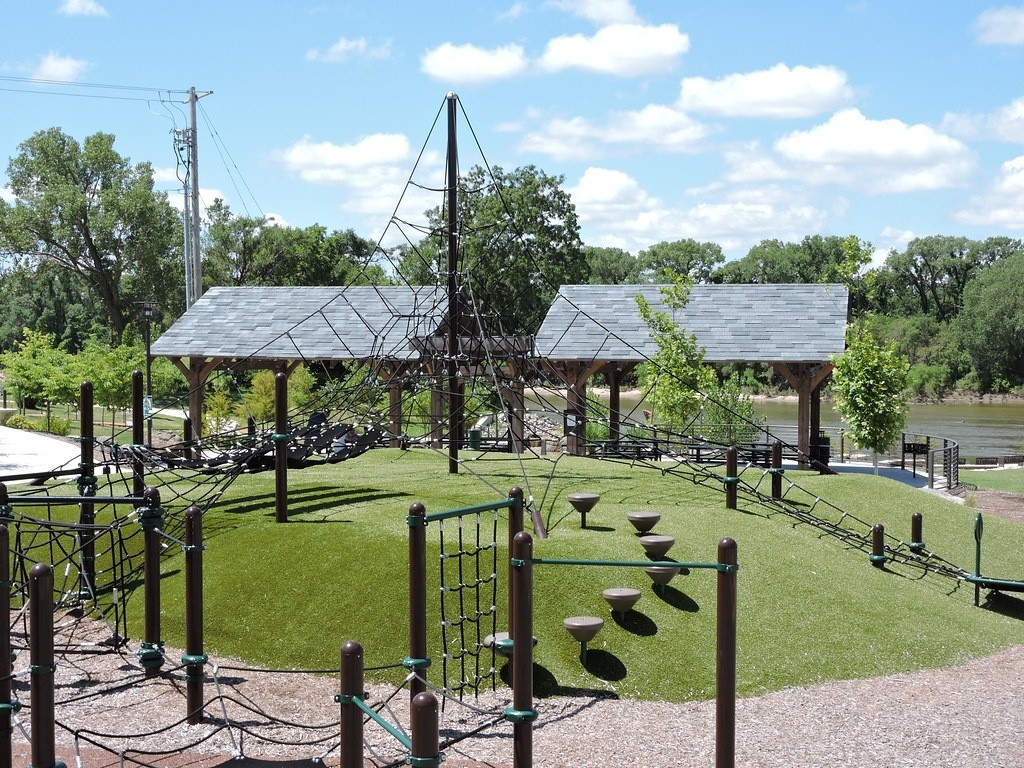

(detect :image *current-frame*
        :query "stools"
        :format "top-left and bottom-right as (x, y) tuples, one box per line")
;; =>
(627, 512), (661, 536)
(640, 535), (676, 560)
(567, 493), (600, 528)
(565, 616), (604, 664)
(603, 588), (641, 623)
(643, 563), (678, 594)
(484, 632), (538, 680)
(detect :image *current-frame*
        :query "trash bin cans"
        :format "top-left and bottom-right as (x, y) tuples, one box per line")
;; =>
(468, 430), (480, 451)
(818, 437), (832, 465)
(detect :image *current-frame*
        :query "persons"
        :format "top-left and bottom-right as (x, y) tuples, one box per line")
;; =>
(334, 427), (369, 453)
(303, 409), (331, 454)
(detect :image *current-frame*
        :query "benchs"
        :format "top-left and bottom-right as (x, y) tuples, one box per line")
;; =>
(583, 444), (645, 457)
